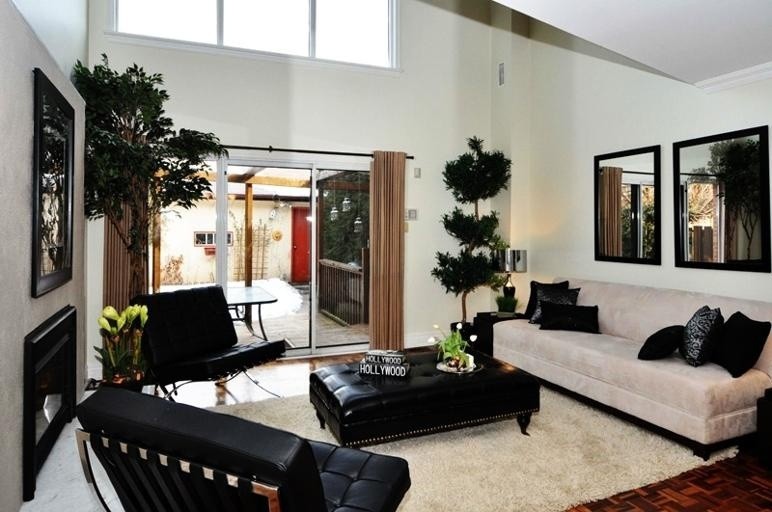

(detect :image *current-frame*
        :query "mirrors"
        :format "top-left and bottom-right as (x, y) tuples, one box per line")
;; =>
(592, 144), (663, 267)
(672, 123), (772, 276)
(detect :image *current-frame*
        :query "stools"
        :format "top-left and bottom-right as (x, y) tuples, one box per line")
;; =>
(308, 346), (543, 452)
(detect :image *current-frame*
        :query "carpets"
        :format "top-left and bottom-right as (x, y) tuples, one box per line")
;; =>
(194, 384), (743, 512)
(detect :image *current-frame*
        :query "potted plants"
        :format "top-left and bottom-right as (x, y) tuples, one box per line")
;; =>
(430, 134), (512, 343)
(495, 296), (518, 318)
(92, 303), (148, 386)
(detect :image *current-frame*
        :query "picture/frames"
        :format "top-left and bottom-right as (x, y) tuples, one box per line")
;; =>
(29, 65), (76, 300)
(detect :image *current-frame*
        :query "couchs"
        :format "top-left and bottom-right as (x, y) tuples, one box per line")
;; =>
(129, 284), (287, 405)
(491, 274), (772, 462)
(74, 385), (412, 512)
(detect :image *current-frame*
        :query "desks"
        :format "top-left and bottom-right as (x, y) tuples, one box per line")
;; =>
(220, 285), (278, 342)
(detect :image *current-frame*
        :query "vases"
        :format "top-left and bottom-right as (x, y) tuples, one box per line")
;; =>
(448, 358), (466, 368)
(103, 365), (146, 388)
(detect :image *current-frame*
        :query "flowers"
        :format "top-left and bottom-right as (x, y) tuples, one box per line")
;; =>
(427, 322), (479, 367)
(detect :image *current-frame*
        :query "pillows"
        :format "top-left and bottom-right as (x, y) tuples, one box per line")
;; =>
(528, 287), (582, 325)
(637, 323), (685, 361)
(721, 310), (772, 378)
(678, 305), (724, 368)
(539, 300), (602, 335)
(521, 280), (570, 320)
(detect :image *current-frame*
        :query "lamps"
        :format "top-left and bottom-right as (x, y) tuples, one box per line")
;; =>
(329, 175), (340, 222)
(353, 171), (365, 234)
(342, 172), (354, 214)
(494, 248), (528, 297)
